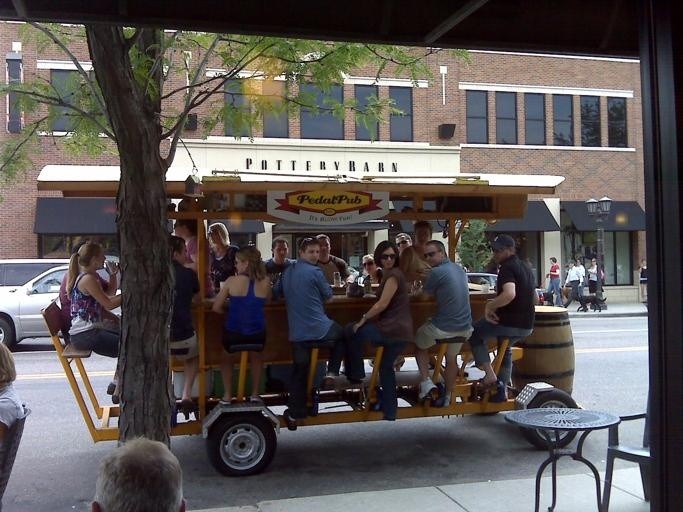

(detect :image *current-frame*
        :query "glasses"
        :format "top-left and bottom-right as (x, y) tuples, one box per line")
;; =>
(381, 254), (396, 260)
(424, 250), (439, 258)
(300, 235), (317, 248)
(397, 241), (408, 247)
(492, 248), (508, 253)
(363, 260), (374, 266)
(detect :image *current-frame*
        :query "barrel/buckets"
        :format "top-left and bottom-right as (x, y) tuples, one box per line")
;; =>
(509, 304), (576, 409)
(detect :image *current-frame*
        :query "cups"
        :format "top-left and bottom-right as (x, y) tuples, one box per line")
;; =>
(481, 284), (489, 292)
(219, 281), (225, 290)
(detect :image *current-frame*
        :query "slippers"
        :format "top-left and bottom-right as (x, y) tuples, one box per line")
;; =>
(475, 377), (498, 391)
(219, 396), (230, 405)
(251, 395), (264, 403)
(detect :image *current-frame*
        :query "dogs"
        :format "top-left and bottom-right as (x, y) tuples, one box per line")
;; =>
(576, 293), (606, 312)
(560, 287), (575, 304)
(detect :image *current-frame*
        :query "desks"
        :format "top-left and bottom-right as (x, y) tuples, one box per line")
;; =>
(503, 406), (620, 512)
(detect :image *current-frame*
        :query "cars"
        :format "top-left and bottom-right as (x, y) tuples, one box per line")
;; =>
(467, 270), (544, 307)
(0, 265), (122, 347)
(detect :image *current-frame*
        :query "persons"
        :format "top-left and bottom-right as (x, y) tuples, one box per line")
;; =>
(91, 437), (186, 512)
(0, 342), (23, 439)
(59, 219), (536, 421)
(527, 257), (647, 306)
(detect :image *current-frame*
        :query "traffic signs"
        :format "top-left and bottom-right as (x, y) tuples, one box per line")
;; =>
(581, 194), (613, 310)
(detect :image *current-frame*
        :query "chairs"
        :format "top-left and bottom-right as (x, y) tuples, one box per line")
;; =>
(0, 405), (34, 501)
(601, 409), (652, 512)
(38, 299), (94, 421)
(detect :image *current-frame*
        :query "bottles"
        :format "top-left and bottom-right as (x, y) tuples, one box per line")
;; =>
(333, 271), (341, 287)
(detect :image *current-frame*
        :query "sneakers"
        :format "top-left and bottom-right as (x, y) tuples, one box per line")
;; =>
(417, 376), (438, 401)
(284, 409), (297, 431)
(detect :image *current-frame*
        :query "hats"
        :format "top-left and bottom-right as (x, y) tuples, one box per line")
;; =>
(487, 234), (514, 248)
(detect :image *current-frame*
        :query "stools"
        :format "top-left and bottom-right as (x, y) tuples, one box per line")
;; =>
(420, 336), (468, 408)
(169, 348), (189, 406)
(226, 343), (265, 403)
(360, 337), (402, 410)
(472, 335), (524, 403)
(295, 339), (335, 412)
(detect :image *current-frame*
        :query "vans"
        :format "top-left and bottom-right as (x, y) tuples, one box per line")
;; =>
(0, 255), (119, 302)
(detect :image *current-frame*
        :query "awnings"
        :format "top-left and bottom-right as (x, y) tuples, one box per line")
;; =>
(560, 200), (646, 231)
(33, 198), (119, 233)
(462, 200), (560, 231)
